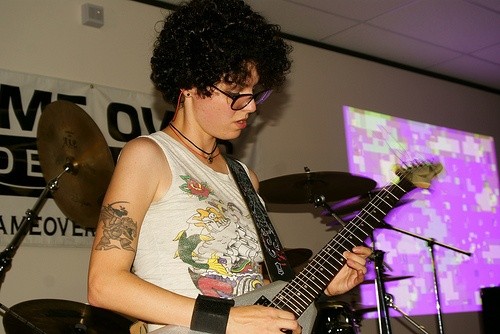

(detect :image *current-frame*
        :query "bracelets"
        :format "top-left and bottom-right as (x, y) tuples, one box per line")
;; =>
(315, 290), (332, 303)
(190, 294), (235, 334)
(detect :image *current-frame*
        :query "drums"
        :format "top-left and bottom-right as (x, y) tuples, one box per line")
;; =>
(1, 297), (137, 334)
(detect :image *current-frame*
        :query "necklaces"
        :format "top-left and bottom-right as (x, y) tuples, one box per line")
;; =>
(169, 122), (220, 163)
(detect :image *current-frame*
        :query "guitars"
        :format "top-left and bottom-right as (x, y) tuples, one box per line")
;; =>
(145, 158), (444, 334)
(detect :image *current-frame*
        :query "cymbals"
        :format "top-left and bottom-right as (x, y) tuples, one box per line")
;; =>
(283, 248), (313, 269)
(257, 170), (378, 206)
(336, 304), (377, 316)
(358, 274), (415, 286)
(36, 99), (115, 233)
(320, 184), (416, 226)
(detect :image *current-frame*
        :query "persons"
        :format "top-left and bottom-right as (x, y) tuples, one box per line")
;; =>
(88, 0), (373, 334)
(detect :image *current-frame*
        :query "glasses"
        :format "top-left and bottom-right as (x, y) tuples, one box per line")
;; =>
(210, 84), (274, 110)
